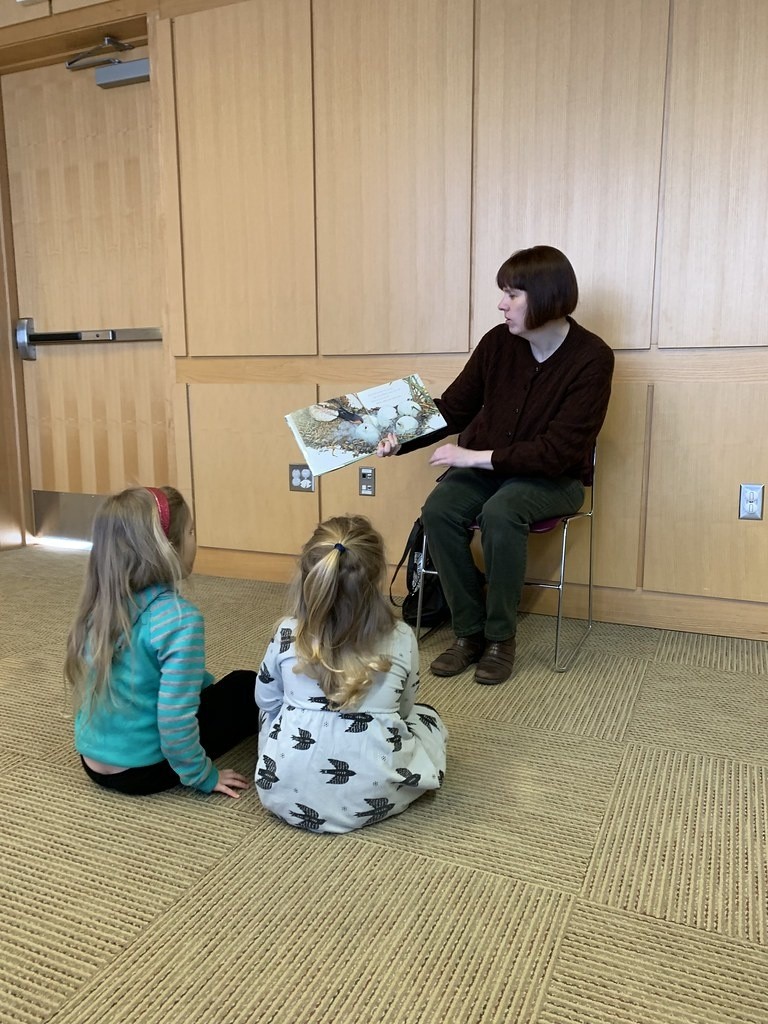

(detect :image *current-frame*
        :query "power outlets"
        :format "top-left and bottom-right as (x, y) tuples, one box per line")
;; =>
(359, 466), (375, 496)
(739, 483), (764, 520)
(289, 464), (315, 492)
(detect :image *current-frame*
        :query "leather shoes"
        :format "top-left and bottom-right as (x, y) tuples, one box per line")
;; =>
(431, 636), (488, 676)
(475, 637), (516, 684)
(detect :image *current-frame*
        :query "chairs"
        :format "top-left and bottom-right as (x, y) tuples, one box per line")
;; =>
(416, 422), (599, 673)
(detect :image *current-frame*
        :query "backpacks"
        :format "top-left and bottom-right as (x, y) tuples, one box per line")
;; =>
(389, 507), (448, 626)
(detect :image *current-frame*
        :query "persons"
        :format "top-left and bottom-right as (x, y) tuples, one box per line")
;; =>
(61, 486), (259, 802)
(254, 515), (449, 835)
(374, 244), (617, 685)
